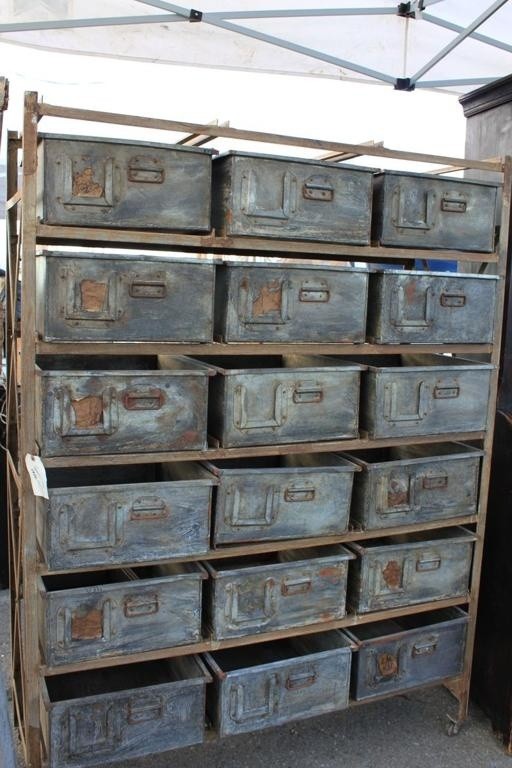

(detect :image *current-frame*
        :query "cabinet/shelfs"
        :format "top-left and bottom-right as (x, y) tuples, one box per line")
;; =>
(5, 91), (511, 767)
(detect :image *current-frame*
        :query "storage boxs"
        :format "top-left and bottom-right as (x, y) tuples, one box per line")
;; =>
(368, 267), (500, 344)
(200, 543), (358, 641)
(201, 632), (365, 739)
(346, 441), (485, 698)
(341, 354), (494, 440)
(40, 561), (210, 668)
(33, 352), (217, 457)
(33, 128), (216, 233)
(197, 453), (362, 550)
(192, 352), (365, 447)
(218, 260), (379, 342)
(373, 165), (502, 254)
(212, 149), (378, 244)
(38, 652), (214, 768)
(36, 461), (219, 578)
(33, 249), (219, 342)
